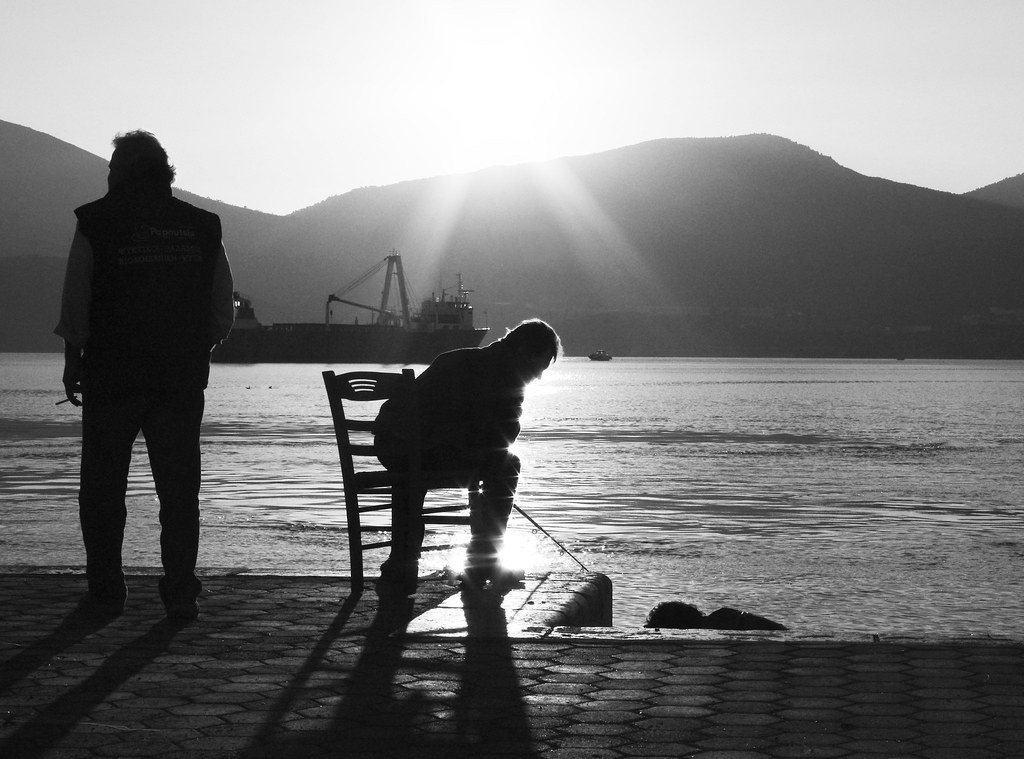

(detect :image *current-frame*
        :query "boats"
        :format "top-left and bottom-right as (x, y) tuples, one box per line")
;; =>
(587, 350), (612, 361)
(209, 246), (490, 365)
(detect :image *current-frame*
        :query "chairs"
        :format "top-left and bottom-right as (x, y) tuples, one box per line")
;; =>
(320, 369), (482, 594)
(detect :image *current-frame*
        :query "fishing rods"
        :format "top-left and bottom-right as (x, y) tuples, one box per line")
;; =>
(512, 502), (592, 574)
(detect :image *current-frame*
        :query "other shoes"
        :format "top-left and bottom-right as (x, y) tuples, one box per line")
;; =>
(166, 601), (199, 619)
(84, 588), (127, 614)
(467, 561), (524, 587)
(380, 556), (419, 576)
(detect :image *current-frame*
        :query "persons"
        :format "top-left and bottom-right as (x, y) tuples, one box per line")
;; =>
(370, 317), (562, 599)
(56, 129), (237, 623)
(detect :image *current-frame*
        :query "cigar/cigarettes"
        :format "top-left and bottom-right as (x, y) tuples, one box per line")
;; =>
(55, 395), (78, 406)
